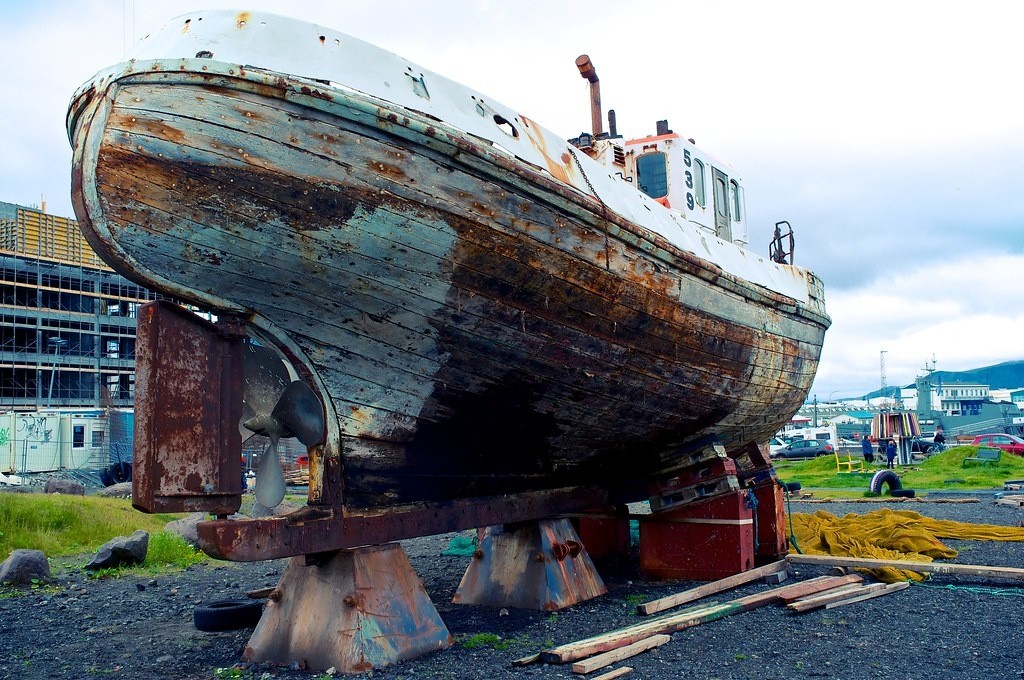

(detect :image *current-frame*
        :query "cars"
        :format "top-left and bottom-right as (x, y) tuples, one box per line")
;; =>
(768, 440), (835, 460)
(768, 437), (793, 455)
(971, 433), (1024, 458)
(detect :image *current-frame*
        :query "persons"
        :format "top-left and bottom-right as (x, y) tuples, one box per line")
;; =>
(825, 421), (829, 427)
(862, 435), (874, 463)
(934, 430), (946, 452)
(848, 419), (852, 424)
(886, 444), (897, 469)
(843, 438), (846, 447)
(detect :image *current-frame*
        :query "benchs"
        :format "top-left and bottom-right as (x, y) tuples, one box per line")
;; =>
(962, 447), (1002, 470)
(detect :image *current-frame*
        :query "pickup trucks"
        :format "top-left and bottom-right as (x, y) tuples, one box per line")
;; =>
(877, 436), (943, 455)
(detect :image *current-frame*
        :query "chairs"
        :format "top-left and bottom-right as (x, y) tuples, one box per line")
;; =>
(835, 450), (865, 473)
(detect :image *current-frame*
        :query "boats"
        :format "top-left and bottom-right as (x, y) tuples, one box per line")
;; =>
(65, 12), (832, 562)
